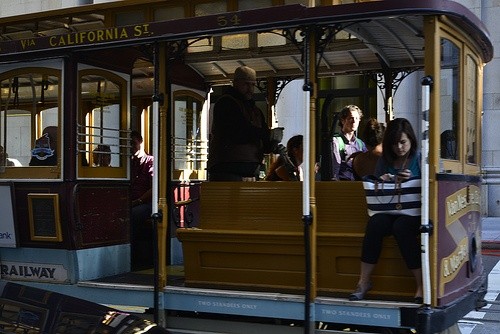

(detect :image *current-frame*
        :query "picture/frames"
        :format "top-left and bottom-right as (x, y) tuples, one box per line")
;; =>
(28, 194), (62, 242)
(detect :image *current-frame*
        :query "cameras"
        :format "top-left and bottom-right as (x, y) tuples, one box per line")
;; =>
(392, 169), (405, 176)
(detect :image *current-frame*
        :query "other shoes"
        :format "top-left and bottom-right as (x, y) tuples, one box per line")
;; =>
(349, 282), (373, 300)
(414, 292), (423, 303)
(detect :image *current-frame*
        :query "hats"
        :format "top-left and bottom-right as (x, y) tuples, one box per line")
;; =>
(233, 66), (257, 82)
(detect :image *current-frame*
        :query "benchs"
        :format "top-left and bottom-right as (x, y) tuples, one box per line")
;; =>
(176, 182), (422, 302)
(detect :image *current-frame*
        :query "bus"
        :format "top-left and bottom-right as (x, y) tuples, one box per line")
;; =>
(0, 0), (494, 334)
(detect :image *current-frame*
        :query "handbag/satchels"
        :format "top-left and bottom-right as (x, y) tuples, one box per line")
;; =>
(362, 179), (421, 217)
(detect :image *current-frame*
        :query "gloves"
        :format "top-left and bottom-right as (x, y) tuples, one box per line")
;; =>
(271, 128), (284, 142)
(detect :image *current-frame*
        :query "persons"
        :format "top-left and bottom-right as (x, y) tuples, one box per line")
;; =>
(93, 144), (111, 167)
(352, 119), (386, 180)
(439, 129), (460, 159)
(28, 126), (89, 167)
(128, 130), (154, 264)
(207, 66), (285, 182)
(326, 104), (369, 182)
(0, 144), (23, 167)
(264, 135), (303, 181)
(348, 118), (422, 305)
